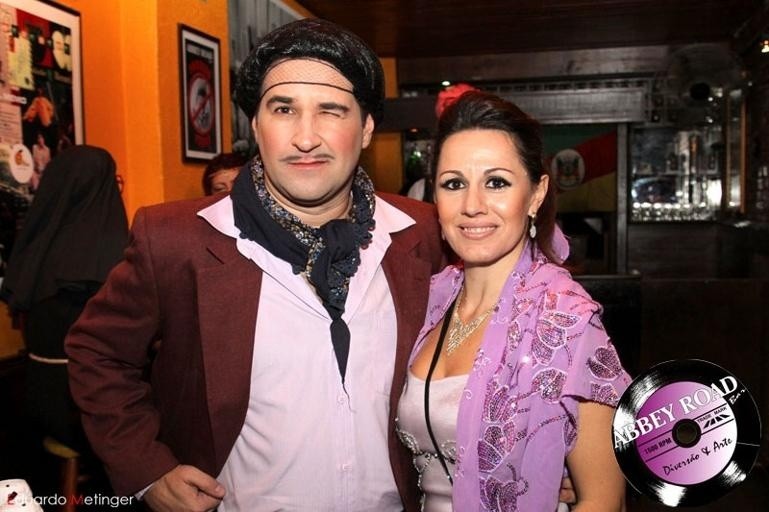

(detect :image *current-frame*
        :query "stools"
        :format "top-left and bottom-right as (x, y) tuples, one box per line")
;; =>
(46, 440), (80, 512)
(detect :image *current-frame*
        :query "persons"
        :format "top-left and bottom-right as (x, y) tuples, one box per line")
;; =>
(30, 133), (52, 192)
(51, 30), (72, 76)
(0, 143), (131, 491)
(200, 152), (250, 196)
(22, 87), (54, 128)
(406, 82), (483, 203)
(64, 14), (578, 512)
(385, 89), (634, 512)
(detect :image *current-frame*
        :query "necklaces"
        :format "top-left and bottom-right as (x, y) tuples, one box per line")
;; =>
(446, 285), (497, 355)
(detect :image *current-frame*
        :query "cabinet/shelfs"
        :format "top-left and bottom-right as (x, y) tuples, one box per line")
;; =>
(629, 67), (744, 222)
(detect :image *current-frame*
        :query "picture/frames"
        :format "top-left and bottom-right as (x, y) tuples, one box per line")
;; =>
(177, 23), (223, 163)
(0, 0), (84, 286)
